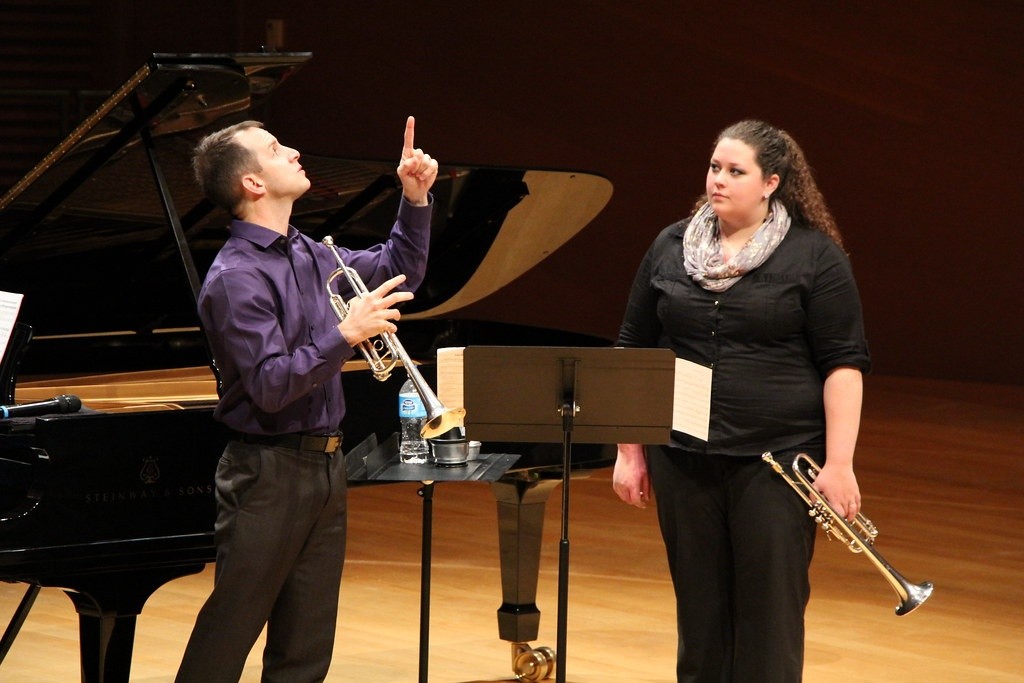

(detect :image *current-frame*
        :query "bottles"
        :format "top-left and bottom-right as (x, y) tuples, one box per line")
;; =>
(399, 365), (429, 463)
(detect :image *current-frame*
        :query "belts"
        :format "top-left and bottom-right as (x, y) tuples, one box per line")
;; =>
(238, 431), (343, 454)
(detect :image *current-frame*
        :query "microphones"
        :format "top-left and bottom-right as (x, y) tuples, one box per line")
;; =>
(0, 394), (82, 420)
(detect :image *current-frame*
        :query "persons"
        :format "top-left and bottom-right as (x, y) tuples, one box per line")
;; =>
(173, 116), (438, 683)
(613, 121), (874, 683)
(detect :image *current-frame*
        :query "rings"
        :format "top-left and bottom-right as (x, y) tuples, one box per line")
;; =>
(848, 502), (857, 508)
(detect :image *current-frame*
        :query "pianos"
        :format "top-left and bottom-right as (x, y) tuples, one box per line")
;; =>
(0, 51), (617, 682)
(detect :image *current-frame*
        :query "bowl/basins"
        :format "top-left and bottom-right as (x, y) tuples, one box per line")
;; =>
(467, 441), (481, 460)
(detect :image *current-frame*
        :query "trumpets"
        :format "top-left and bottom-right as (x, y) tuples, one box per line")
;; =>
(322, 233), (465, 441)
(763, 447), (934, 615)
